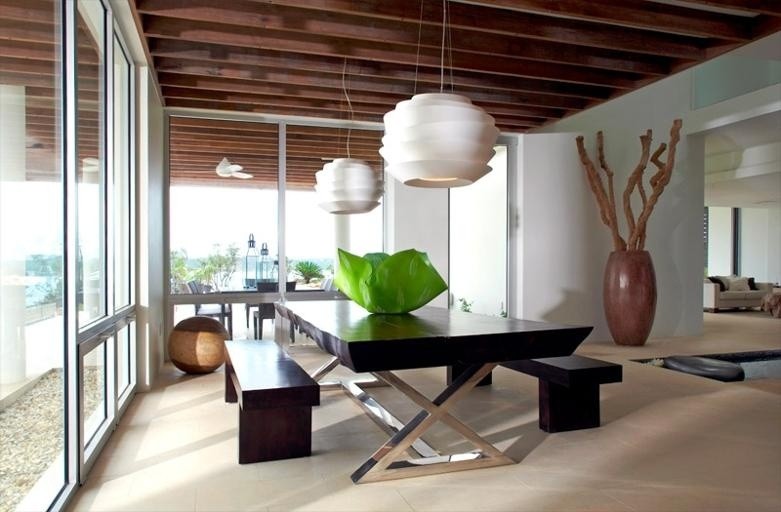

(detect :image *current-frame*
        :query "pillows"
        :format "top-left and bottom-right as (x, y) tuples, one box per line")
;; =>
(708, 274), (759, 291)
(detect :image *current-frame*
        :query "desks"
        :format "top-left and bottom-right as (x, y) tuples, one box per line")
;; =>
(271, 298), (595, 486)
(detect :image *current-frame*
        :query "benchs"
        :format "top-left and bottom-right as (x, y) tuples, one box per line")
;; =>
(446, 353), (623, 434)
(222, 337), (321, 465)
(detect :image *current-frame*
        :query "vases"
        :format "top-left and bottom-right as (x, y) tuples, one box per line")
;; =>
(602, 249), (657, 346)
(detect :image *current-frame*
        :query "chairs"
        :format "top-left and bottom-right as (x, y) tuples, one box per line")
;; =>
(184, 277), (340, 342)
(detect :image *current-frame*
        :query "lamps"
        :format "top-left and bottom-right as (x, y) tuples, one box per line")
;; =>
(313, 57), (386, 215)
(378, 1), (500, 189)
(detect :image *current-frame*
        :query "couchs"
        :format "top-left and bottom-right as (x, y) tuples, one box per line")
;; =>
(703, 278), (774, 314)
